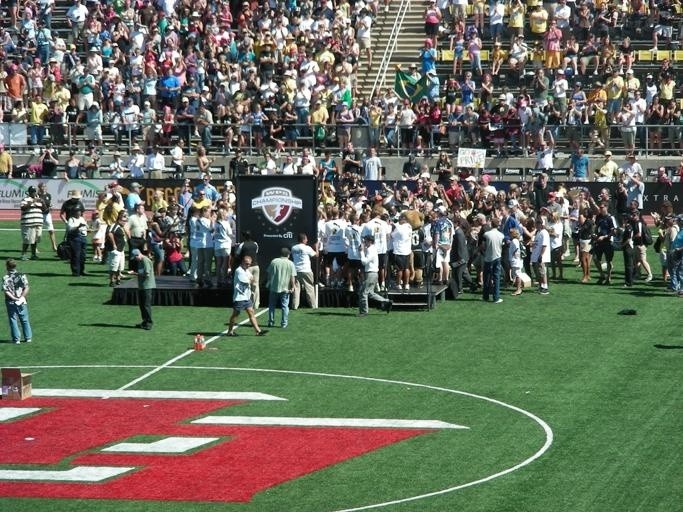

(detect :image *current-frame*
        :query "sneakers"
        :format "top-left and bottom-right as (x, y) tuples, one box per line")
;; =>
(110, 270), (133, 287)
(256, 329), (268, 335)
(375, 282), (424, 292)
(563, 251), (611, 285)
(14, 340), (20, 344)
(622, 272), (654, 287)
(72, 272), (87, 278)
(663, 276), (683, 295)
(470, 281), (522, 303)
(534, 286), (549, 295)
(136, 322), (153, 330)
(432, 280), (447, 285)
(94, 256), (105, 264)
(187, 270), (232, 287)
(25, 339), (31, 342)
(19, 255), (40, 261)
(227, 331), (239, 336)
(319, 277), (363, 292)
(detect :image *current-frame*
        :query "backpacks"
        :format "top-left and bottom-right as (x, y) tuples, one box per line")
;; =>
(635, 220), (653, 245)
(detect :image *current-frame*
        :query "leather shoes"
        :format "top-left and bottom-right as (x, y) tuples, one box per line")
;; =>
(356, 311), (368, 317)
(387, 300), (393, 313)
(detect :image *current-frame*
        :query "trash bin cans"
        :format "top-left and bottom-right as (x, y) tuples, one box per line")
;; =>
(449, 126), (464, 146)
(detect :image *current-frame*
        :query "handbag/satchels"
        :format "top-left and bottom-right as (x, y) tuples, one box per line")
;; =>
(654, 237), (661, 252)
(521, 241), (528, 258)
(58, 241), (72, 259)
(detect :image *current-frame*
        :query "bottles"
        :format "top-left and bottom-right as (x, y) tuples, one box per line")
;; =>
(193, 333), (205, 351)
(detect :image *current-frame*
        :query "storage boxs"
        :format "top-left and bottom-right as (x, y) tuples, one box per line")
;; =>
(2, 367), (40, 400)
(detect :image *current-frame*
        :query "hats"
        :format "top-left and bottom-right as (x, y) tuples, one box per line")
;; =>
(508, 199), (519, 210)
(110, 181), (118, 188)
(98, 192), (106, 199)
(10, 0), (369, 152)
(29, 185), (36, 192)
(547, 193), (557, 198)
(433, 199), (448, 215)
(448, 174), (476, 182)
(155, 212), (162, 218)
(403, 0), (670, 157)
(70, 189), (82, 199)
(664, 212), (683, 221)
(92, 209), (98, 214)
(129, 249), (139, 261)
(130, 182), (142, 189)
(224, 181), (232, 186)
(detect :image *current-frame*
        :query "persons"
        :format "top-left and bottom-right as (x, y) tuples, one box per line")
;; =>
(266, 248), (297, 327)
(1, 258), (32, 343)
(131, 248), (157, 330)
(1, 0), (682, 315)
(227, 256), (269, 336)
